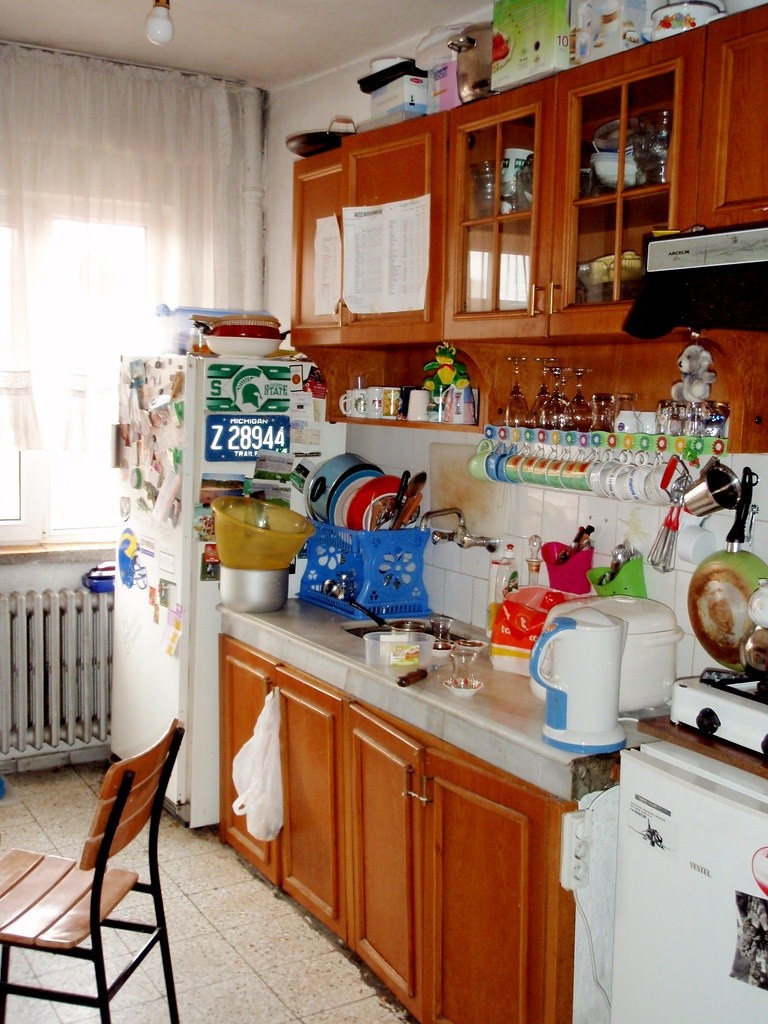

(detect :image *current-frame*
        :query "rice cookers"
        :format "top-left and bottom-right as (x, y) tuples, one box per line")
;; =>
(530, 595), (684, 712)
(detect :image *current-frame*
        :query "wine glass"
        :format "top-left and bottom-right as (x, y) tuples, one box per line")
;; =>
(565, 367), (592, 432)
(504, 358), (577, 432)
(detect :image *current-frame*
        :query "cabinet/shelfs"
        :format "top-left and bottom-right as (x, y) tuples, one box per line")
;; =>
(216, 634), (578, 1024)
(290, 3), (768, 455)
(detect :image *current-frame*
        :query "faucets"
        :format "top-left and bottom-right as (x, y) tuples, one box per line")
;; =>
(419, 506), (466, 534)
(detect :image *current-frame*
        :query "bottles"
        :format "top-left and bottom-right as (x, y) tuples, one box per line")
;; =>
(526, 535), (543, 588)
(487, 560), (499, 638)
(495, 544), (518, 613)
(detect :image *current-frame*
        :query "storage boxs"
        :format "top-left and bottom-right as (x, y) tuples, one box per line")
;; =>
(357, 60), (428, 94)
(157, 304), (272, 355)
(356, 111), (422, 133)
(370, 75), (428, 115)
(569, 0), (648, 66)
(489, 0), (568, 91)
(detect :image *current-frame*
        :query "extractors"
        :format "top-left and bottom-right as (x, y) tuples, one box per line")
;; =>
(622, 220), (768, 339)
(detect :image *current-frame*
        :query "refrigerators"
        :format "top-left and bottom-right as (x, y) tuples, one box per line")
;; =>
(111, 354), (346, 829)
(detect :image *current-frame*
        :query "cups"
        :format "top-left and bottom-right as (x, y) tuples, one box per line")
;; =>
(190, 328), (210, 356)
(467, 439), (741, 565)
(589, 393), (731, 438)
(338, 386), (476, 425)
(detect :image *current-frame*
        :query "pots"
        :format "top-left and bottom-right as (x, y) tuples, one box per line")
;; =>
(308, 454), (376, 523)
(350, 600), (426, 632)
(689, 466), (768, 671)
(219, 563), (289, 613)
(193, 321), (292, 340)
(284, 3), (727, 158)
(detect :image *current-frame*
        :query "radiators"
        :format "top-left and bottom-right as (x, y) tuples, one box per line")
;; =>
(0, 586), (112, 755)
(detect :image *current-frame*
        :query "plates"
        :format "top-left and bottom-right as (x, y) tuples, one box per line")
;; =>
(328, 471), (421, 529)
(433, 640), (489, 698)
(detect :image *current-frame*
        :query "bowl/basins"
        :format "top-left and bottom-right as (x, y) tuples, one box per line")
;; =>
(470, 111), (672, 286)
(203, 336), (283, 359)
(211, 496), (315, 569)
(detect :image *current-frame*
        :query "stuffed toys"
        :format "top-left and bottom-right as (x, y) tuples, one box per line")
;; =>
(669, 344), (716, 405)
(423, 344), (470, 396)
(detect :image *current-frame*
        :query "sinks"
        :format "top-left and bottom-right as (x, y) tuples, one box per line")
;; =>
(345, 620), (467, 656)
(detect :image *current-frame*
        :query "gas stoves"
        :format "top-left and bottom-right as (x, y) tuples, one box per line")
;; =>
(669, 668), (768, 765)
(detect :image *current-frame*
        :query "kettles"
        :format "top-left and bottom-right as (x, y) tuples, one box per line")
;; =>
(529, 607), (628, 754)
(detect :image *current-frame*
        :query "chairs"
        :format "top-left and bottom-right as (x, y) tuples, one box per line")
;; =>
(0, 717), (186, 1024)
